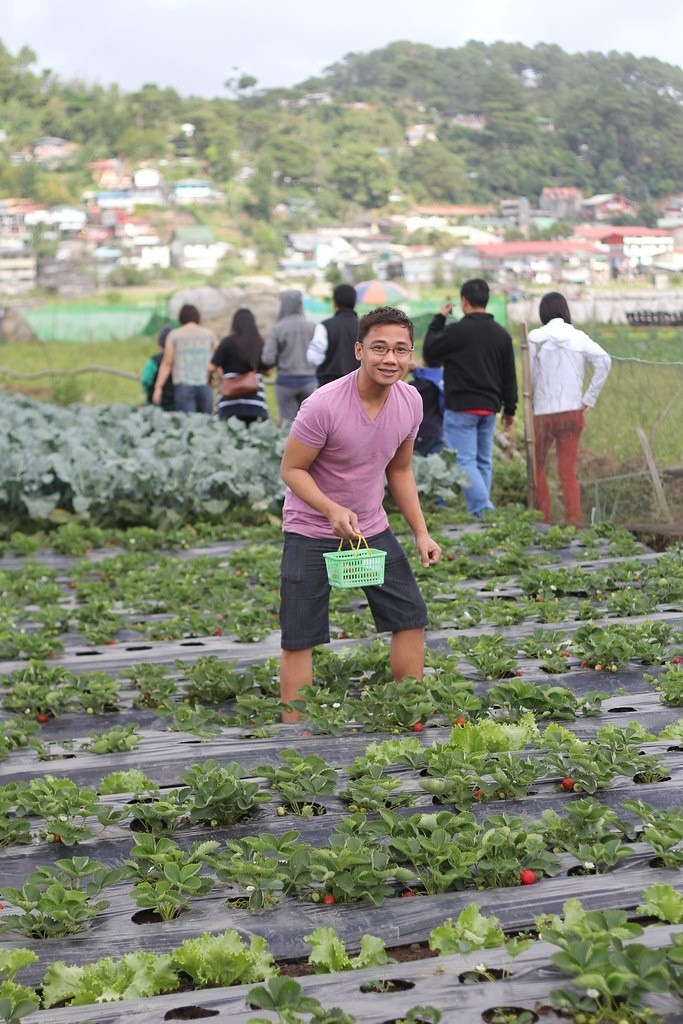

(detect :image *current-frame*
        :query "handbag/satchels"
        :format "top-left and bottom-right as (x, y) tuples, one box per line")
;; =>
(221, 370), (259, 399)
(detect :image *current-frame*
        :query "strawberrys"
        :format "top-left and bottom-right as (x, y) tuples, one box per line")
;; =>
(36, 547), (681, 907)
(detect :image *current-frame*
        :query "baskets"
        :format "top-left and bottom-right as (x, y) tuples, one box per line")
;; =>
(323, 534), (387, 589)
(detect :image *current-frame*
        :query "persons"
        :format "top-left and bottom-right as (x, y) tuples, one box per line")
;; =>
(260, 289), (321, 425)
(140, 305), (218, 413)
(406, 354), (443, 458)
(211, 307), (269, 429)
(527, 291), (612, 534)
(282, 307), (443, 720)
(306, 284), (360, 387)
(422, 278), (518, 516)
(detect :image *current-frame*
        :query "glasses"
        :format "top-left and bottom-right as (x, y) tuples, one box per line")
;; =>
(360, 344), (414, 356)
